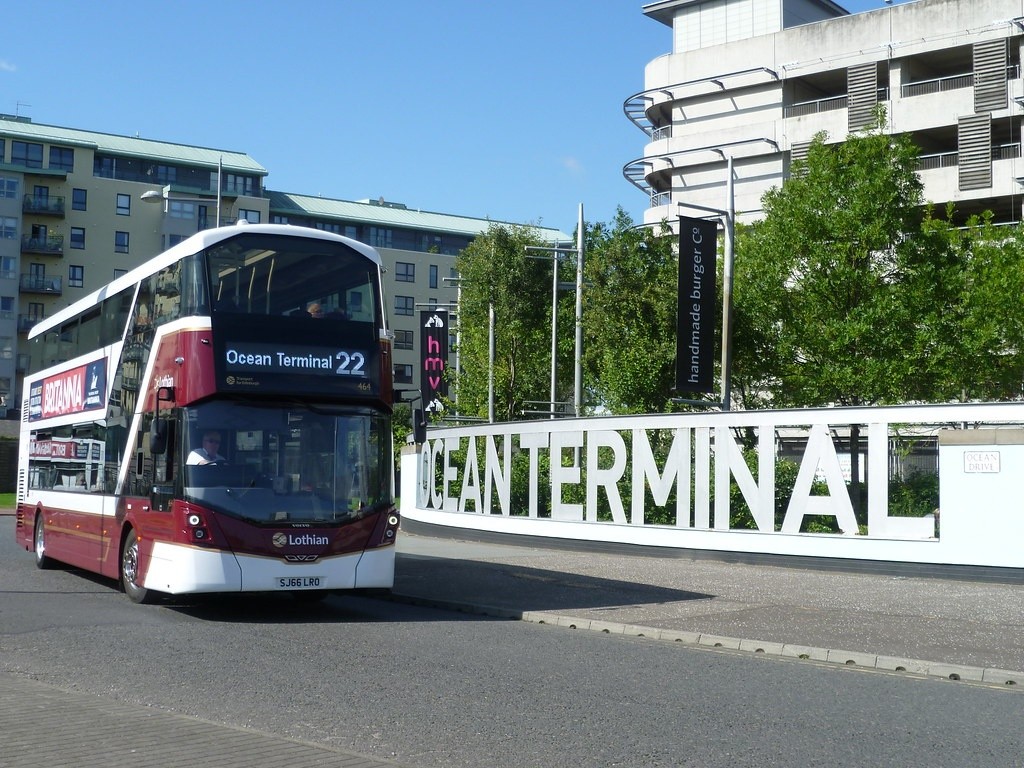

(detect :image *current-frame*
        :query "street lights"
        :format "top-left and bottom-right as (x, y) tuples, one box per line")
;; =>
(140, 159), (221, 231)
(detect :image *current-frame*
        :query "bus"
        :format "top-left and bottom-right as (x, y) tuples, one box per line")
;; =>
(15, 224), (428, 605)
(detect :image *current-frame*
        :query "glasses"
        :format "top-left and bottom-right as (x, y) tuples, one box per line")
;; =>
(310, 309), (323, 314)
(205, 439), (221, 444)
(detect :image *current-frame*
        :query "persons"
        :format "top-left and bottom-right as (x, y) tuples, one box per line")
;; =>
(186, 431), (230, 466)
